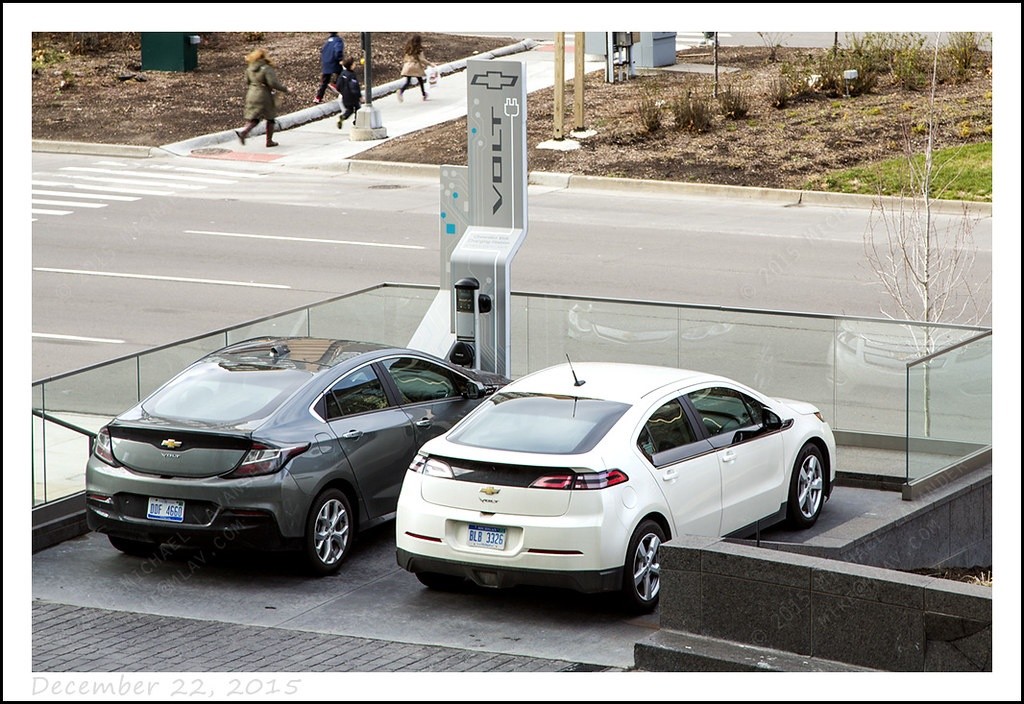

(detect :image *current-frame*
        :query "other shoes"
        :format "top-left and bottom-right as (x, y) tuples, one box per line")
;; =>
(313, 97), (325, 104)
(396, 89), (403, 102)
(328, 82), (340, 94)
(423, 92), (428, 101)
(338, 115), (344, 129)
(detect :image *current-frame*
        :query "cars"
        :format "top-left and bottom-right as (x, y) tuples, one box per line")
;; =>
(83, 333), (529, 576)
(394, 360), (838, 612)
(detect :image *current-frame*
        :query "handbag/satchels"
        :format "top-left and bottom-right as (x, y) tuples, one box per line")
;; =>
(427, 64), (440, 87)
(339, 94), (347, 114)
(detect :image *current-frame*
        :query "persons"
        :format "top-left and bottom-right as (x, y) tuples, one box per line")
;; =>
(397, 34), (435, 103)
(336, 56), (366, 129)
(234, 48), (294, 147)
(313, 32), (344, 104)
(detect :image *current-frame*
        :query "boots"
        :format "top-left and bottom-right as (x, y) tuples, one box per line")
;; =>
(266, 124), (278, 147)
(235, 122), (256, 144)
